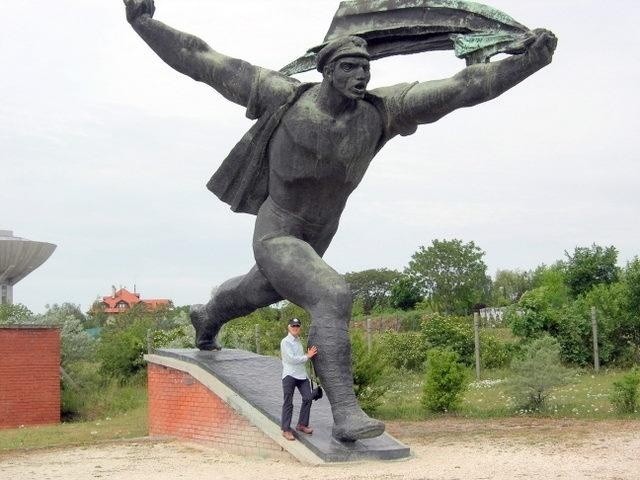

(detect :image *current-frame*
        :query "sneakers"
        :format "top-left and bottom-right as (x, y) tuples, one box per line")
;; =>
(282, 432), (294, 440)
(296, 424), (313, 434)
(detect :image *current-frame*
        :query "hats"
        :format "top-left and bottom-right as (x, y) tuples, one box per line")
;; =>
(288, 318), (302, 327)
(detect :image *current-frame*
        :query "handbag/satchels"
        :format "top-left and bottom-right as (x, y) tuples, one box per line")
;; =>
(310, 385), (323, 401)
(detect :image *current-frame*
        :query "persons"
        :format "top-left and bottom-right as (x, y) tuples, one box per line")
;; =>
(124, 1), (556, 441)
(279, 318), (318, 441)
